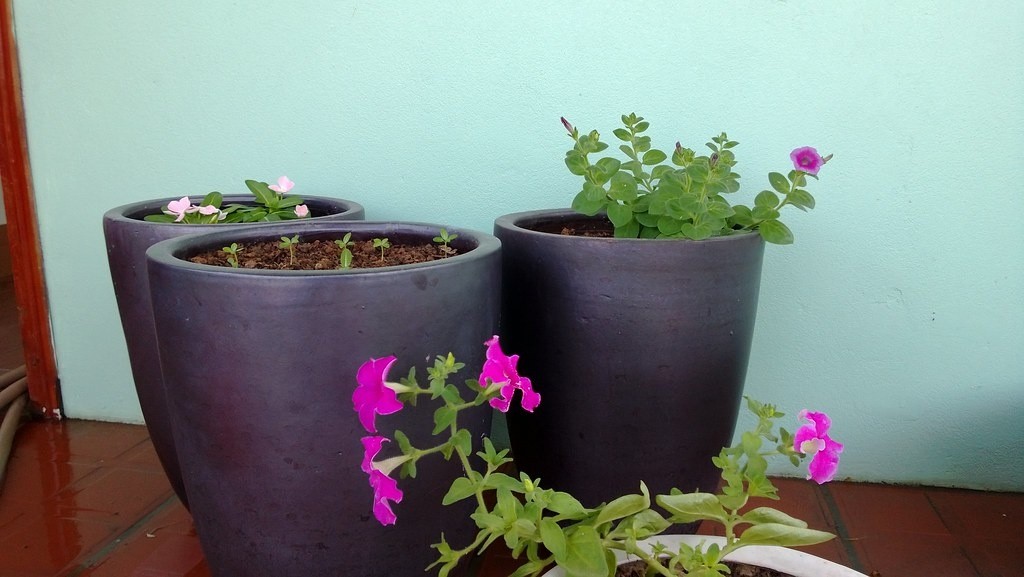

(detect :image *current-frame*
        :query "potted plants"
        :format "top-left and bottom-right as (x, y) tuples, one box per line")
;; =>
(144, 222), (503, 576)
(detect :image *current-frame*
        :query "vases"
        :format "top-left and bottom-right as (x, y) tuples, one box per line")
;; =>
(102, 192), (366, 517)
(542, 535), (868, 577)
(494, 208), (764, 535)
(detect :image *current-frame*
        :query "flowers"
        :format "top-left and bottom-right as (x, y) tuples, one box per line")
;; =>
(144, 175), (312, 224)
(559, 112), (832, 244)
(351, 335), (844, 577)
